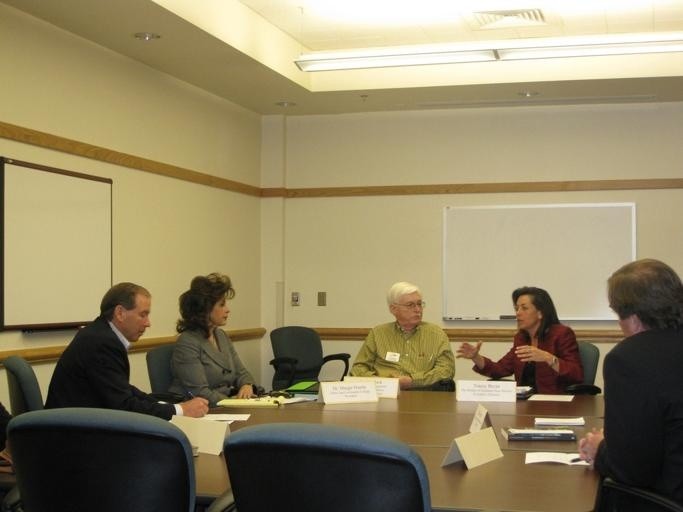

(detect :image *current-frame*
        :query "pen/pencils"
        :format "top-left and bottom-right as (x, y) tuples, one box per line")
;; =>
(187, 391), (195, 399)
(571, 455), (588, 462)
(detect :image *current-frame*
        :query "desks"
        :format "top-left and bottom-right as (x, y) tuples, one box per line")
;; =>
(195, 388), (606, 511)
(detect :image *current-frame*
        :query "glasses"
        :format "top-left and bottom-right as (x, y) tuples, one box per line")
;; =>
(394, 301), (425, 310)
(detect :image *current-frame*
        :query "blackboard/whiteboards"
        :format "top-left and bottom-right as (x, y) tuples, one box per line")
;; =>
(500, 315), (516, 320)
(442, 202), (636, 322)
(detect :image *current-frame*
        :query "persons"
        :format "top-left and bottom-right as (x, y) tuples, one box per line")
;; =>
(45, 282), (209, 420)
(347, 281), (455, 391)
(456, 287), (585, 394)
(169, 273), (261, 400)
(578, 257), (681, 510)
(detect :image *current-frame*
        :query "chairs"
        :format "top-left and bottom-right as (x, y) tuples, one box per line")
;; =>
(565, 340), (602, 395)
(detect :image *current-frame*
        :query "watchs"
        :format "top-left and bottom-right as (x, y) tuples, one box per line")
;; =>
(549, 354), (558, 368)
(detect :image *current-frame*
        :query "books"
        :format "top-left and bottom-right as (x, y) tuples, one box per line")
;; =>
(285, 380), (320, 395)
(499, 426), (576, 441)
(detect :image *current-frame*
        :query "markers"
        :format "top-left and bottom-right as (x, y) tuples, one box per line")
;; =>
(455, 318), (462, 320)
